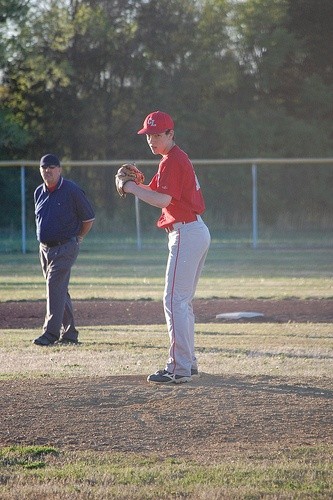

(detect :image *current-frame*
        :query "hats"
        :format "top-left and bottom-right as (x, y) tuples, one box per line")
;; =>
(137, 111), (175, 135)
(40, 154), (60, 166)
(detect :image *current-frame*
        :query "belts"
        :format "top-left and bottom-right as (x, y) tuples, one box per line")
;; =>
(163, 217), (198, 233)
(41, 236), (77, 247)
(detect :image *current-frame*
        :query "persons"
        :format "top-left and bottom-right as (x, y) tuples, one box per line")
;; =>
(115, 110), (211, 385)
(34, 154), (96, 346)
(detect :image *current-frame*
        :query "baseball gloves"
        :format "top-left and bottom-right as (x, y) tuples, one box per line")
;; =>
(114, 163), (144, 199)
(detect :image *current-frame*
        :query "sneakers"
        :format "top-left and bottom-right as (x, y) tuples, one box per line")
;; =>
(147, 373), (192, 385)
(54, 336), (78, 345)
(155, 368), (199, 377)
(34, 334), (53, 346)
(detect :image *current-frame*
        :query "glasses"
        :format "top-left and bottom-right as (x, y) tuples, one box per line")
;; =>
(42, 165), (56, 169)
